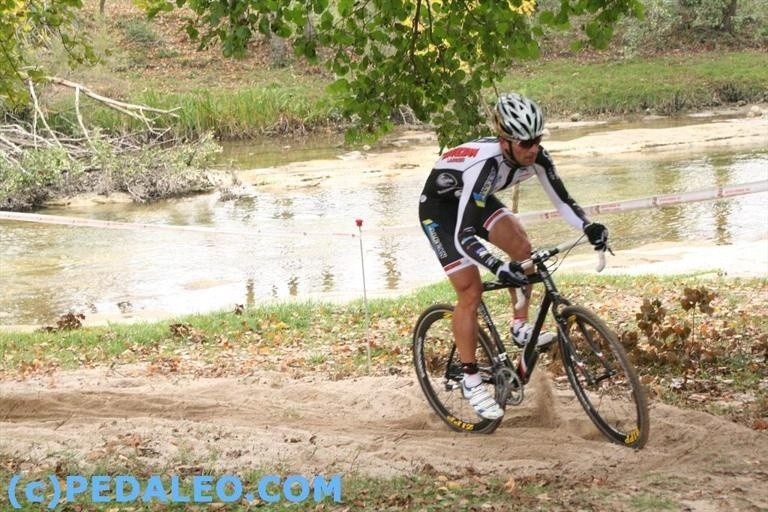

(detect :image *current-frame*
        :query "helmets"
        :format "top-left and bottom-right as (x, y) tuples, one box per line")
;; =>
(492, 92), (545, 141)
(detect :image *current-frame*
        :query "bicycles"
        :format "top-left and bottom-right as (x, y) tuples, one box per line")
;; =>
(412, 236), (650, 451)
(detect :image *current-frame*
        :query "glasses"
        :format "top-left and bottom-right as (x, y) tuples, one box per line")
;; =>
(517, 134), (543, 149)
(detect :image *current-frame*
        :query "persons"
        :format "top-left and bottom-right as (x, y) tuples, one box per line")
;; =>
(418, 94), (609, 422)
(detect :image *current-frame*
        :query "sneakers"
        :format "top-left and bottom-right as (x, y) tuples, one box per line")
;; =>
(509, 322), (559, 349)
(462, 380), (505, 420)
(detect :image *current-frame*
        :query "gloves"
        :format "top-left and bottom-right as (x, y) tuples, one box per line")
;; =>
(497, 262), (530, 288)
(583, 221), (609, 252)
